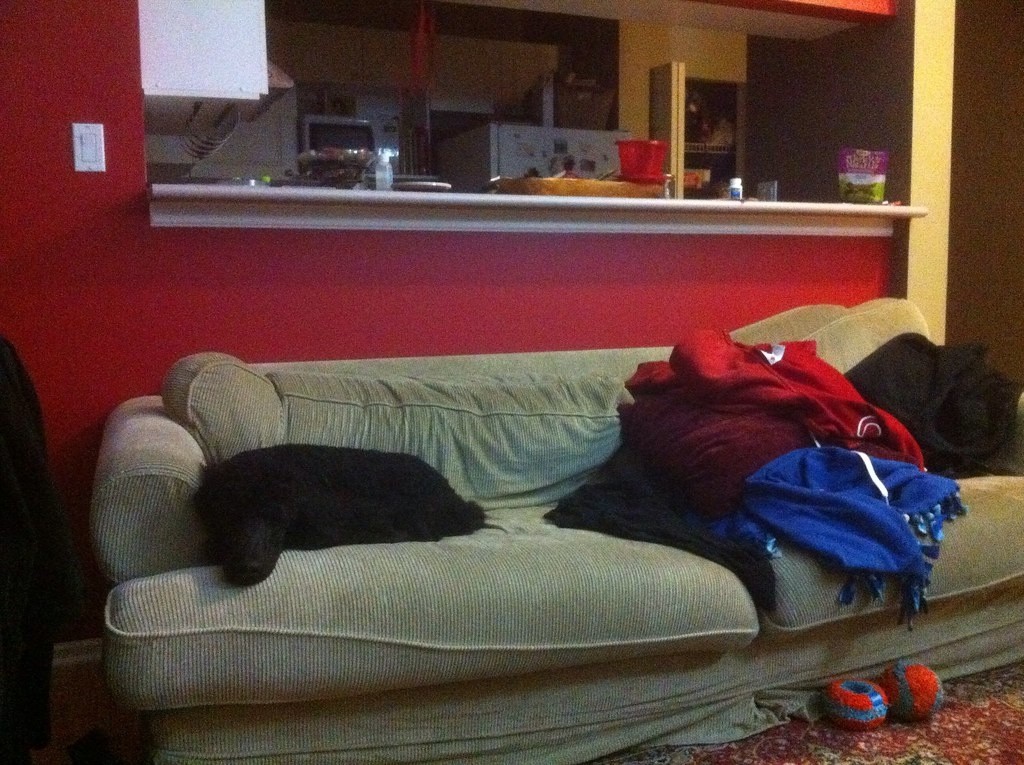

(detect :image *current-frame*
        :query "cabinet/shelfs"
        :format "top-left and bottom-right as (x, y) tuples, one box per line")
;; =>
(681, 76), (748, 203)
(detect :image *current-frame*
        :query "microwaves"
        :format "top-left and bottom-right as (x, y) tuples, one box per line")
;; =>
(303, 115), (400, 176)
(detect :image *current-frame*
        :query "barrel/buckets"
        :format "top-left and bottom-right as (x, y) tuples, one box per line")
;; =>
(614, 140), (669, 184)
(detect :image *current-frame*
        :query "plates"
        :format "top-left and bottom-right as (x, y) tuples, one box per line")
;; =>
(392, 181), (452, 192)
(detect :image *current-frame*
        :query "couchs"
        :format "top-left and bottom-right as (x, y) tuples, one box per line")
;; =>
(89, 298), (1024, 765)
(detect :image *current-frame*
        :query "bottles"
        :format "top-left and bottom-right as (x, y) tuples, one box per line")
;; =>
(729, 179), (744, 199)
(375, 154), (394, 191)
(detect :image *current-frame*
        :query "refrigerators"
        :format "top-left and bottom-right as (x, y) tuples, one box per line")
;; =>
(450, 121), (633, 197)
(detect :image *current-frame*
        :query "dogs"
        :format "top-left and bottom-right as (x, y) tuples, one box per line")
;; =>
(189, 443), (495, 589)
